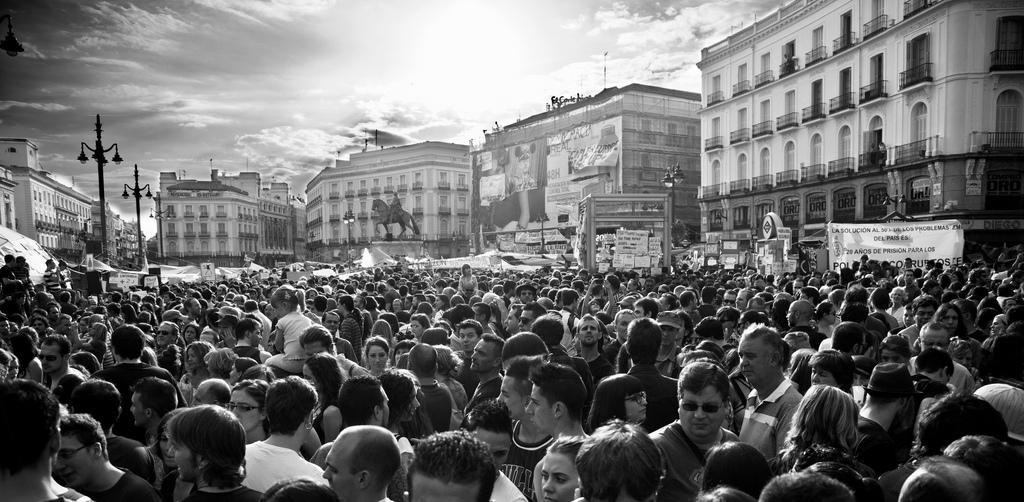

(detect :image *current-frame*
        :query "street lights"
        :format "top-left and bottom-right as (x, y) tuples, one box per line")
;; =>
(660, 161), (686, 274)
(535, 211), (550, 254)
(122, 162), (154, 270)
(342, 209), (355, 274)
(75, 112), (126, 271)
(148, 192), (172, 266)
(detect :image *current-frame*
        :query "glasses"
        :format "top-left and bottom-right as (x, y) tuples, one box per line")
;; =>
(154, 329), (169, 336)
(626, 392), (648, 403)
(723, 299), (735, 304)
(61, 446), (85, 460)
(37, 355), (57, 362)
(681, 398), (723, 413)
(518, 316), (532, 325)
(223, 401), (258, 413)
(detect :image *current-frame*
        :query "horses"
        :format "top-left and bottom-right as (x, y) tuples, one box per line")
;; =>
(370, 198), (418, 239)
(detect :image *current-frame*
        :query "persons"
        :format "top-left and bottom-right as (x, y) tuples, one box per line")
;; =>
(1, 241), (1022, 502)
(386, 192), (402, 221)
(472, 144), (538, 252)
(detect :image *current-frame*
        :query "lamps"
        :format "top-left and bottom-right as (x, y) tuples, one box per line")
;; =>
(882, 194), (907, 205)
(715, 212), (727, 224)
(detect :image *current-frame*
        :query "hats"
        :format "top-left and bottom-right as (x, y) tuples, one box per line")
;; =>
(976, 382), (1024, 442)
(853, 335), (916, 395)
(617, 296), (637, 308)
(163, 310), (189, 322)
(214, 306), (238, 325)
(516, 283), (536, 297)
(658, 312), (683, 331)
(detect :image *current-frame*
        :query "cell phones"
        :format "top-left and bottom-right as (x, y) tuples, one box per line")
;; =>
(596, 279), (603, 284)
